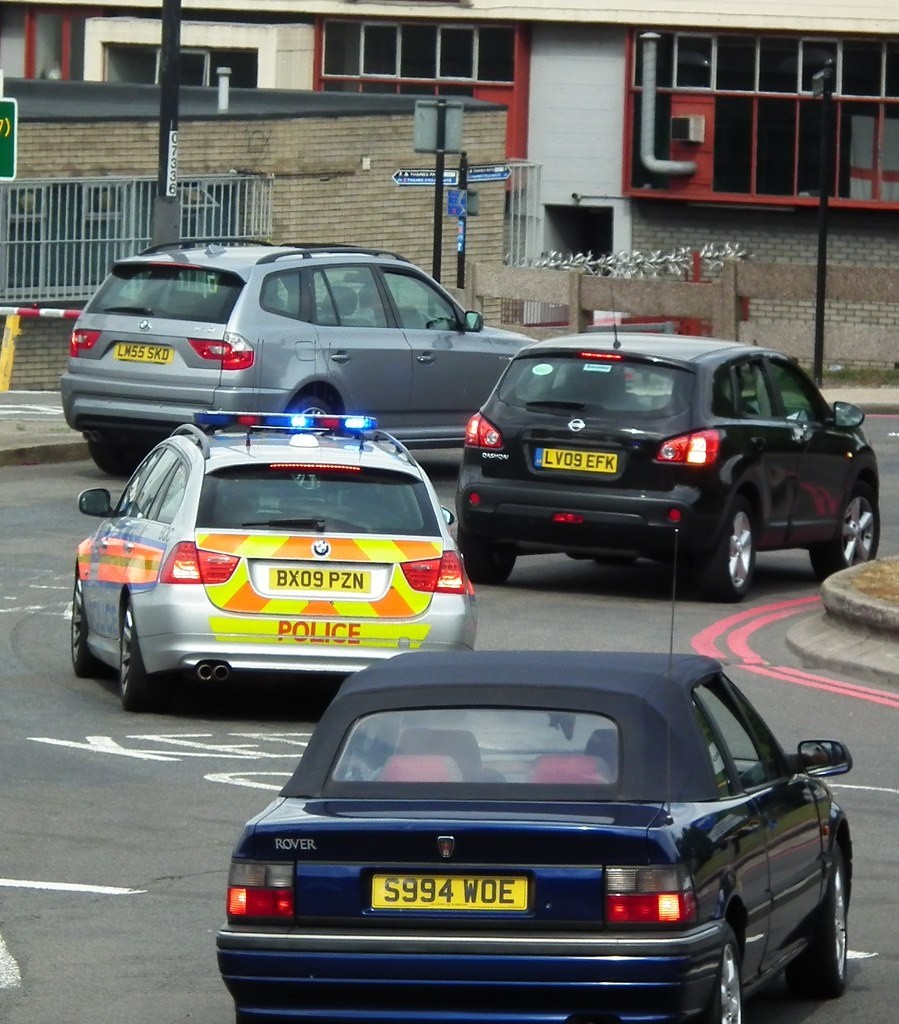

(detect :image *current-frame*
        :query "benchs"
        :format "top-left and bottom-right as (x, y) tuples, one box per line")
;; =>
(226, 490), (388, 521)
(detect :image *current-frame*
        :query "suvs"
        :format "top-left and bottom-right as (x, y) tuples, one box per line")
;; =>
(58, 235), (544, 477)
(453, 329), (883, 603)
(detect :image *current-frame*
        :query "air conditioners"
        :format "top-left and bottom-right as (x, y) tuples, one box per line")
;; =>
(669, 115), (705, 142)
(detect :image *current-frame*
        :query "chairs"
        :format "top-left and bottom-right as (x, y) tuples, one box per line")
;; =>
(383, 729), (620, 785)
(321, 285), (373, 326)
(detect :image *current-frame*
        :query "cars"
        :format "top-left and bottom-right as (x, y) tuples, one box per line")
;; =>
(215, 649), (855, 1024)
(69, 409), (479, 715)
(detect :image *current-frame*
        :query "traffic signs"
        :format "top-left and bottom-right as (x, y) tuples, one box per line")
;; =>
(391, 166), (459, 188)
(446, 189), (471, 218)
(466, 165), (514, 184)
